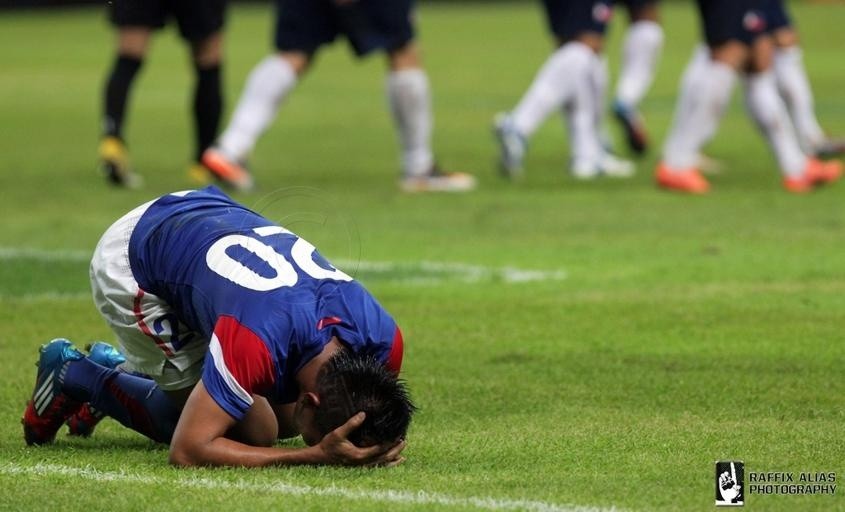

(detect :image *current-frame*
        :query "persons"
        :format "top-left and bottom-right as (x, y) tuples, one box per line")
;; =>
(675, 0), (844, 174)
(95, 1), (233, 191)
(20, 187), (415, 467)
(655, 0), (844, 193)
(608, 0), (665, 157)
(491, 0), (636, 182)
(197, 1), (478, 193)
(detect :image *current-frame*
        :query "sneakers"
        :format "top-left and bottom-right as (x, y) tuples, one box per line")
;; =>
(20, 338), (126, 448)
(495, 113), (527, 173)
(569, 101), (720, 192)
(780, 140), (845, 192)
(99, 136), (128, 185)
(397, 169), (470, 192)
(190, 145), (252, 191)
